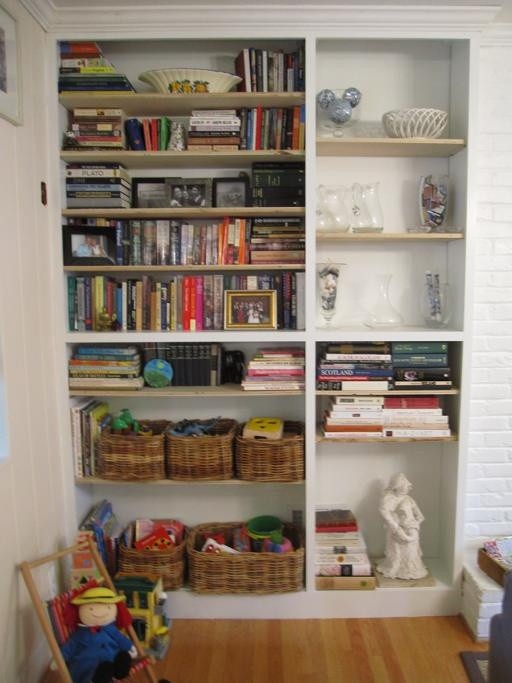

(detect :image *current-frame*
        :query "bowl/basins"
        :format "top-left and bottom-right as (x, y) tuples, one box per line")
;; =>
(137, 66), (244, 93)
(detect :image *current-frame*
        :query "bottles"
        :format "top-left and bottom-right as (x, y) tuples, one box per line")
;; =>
(316, 180), (382, 236)
(365, 275), (405, 330)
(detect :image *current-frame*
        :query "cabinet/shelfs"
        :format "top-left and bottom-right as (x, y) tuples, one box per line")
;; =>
(47, 26), (475, 625)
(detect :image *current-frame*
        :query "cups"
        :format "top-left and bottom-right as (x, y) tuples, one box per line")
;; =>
(417, 282), (454, 330)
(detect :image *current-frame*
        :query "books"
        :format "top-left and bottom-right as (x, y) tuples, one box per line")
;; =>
(322, 343), (456, 439)
(71, 398), (108, 478)
(316, 510), (371, 576)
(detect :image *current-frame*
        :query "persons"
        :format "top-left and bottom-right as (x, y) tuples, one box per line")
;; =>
(372, 471), (429, 577)
(50, 588), (137, 683)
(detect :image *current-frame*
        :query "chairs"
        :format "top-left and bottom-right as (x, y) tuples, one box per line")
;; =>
(485, 571), (511, 682)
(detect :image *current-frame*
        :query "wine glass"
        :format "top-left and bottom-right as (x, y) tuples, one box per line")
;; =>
(317, 263), (339, 329)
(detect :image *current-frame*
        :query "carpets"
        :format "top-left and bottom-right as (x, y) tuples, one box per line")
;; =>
(460, 651), (489, 682)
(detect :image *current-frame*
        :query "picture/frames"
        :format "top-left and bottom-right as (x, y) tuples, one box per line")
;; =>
(1, 3), (27, 126)
(222, 287), (277, 332)
